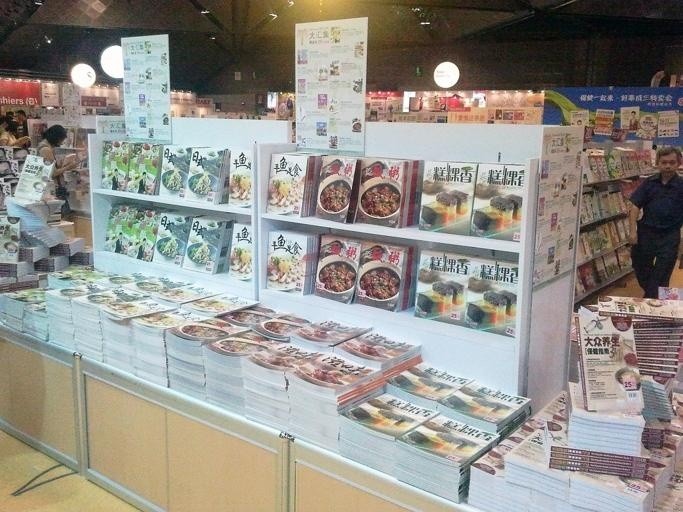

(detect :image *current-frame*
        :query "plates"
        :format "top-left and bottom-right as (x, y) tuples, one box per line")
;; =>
(223, 163), (311, 296)
(215, 304), (407, 389)
(341, 360), (532, 474)
(410, 202), (521, 335)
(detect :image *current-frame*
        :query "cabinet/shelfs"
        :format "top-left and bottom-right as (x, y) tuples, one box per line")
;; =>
(572, 151), (657, 304)
(1, 325), (77, 512)
(75, 354), (286, 510)
(26, 114), (585, 419)
(288, 436), (477, 512)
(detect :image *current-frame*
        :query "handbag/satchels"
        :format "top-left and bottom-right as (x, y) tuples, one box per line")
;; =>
(55, 187), (70, 215)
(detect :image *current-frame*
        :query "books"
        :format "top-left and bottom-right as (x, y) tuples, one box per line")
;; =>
(1, 145), (94, 294)
(581, 176), (649, 224)
(2, 270), (263, 388)
(338, 362), (532, 504)
(578, 218), (636, 262)
(466, 292), (682, 512)
(165, 307), (425, 453)
(265, 151), (524, 242)
(574, 246), (633, 296)
(266, 229), (519, 339)
(100, 138), (253, 209)
(582, 149), (655, 185)
(104, 202), (254, 282)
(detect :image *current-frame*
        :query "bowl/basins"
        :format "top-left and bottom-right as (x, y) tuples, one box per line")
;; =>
(59, 169), (217, 328)
(319, 174), (401, 310)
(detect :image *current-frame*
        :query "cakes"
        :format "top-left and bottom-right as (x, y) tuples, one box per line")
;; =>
(417, 281), (517, 326)
(420, 189), (524, 233)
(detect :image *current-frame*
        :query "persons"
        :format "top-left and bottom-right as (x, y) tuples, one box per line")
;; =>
(630, 110), (638, 130)
(1, 109), (78, 221)
(626, 147), (682, 299)
